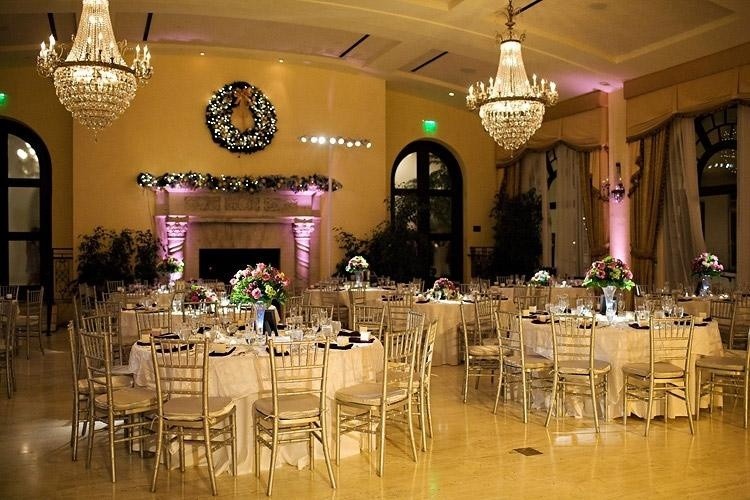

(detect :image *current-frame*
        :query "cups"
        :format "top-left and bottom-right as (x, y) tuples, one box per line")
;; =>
(177, 313), (342, 351)
(555, 295), (683, 325)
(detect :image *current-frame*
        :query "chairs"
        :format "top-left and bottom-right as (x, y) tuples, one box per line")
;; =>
(492, 309), (553, 424)
(149, 332), (237, 496)
(620, 315), (695, 436)
(544, 312), (611, 434)
(79, 330), (168, 484)
(693, 325), (749, 429)
(67, 271), (750, 335)
(1, 279), (19, 300)
(710, 298), (739, 348)
(334, 327), (418, 477)
(251, 335), (336, 496)
(459, 300), (511, 404)
(79, 313), (132, 437)
(474, 291), (508, 390)
(67, 319), (134, 461)
(383, 311), (426, 410)
(15, 286), (45, 360)
(376, 319), (438, 452)
(2, 302), (17, 398)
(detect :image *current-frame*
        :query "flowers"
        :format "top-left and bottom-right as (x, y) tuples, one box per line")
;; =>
(155, 252), (184, 276)
(228, 260), (294, 310)
(344, 255), (370, 273)
(692, 251), (727, 275)
(581, 258), (633, 290)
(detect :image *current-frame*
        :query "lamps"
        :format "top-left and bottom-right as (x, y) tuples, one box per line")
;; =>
(607, 161), (627, 205)
(295, 134), (372, 150)
(33, 0), (155, 135)
(466, 0), (559, 159)
